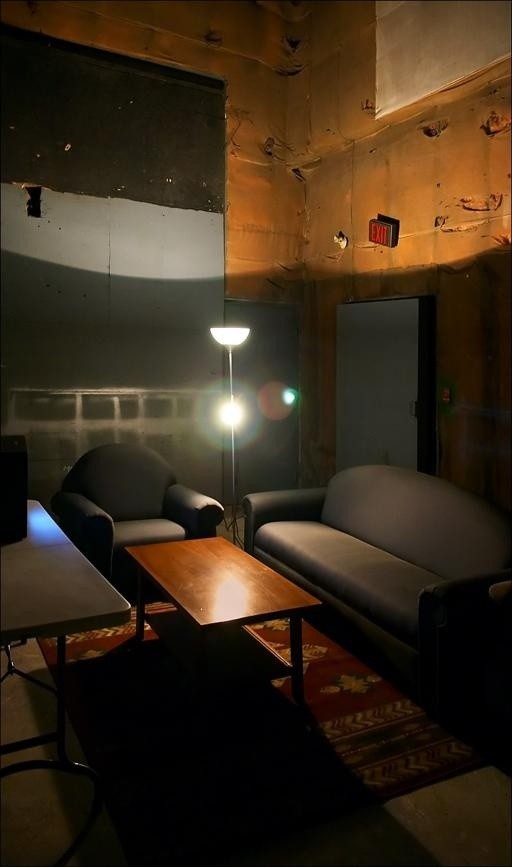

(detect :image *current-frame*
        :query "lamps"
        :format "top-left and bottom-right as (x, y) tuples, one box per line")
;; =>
(209, 323), (252, 543)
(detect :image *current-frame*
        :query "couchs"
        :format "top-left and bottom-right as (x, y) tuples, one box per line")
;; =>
(48, 443), (225, 580)
(241, 464), (512, 680)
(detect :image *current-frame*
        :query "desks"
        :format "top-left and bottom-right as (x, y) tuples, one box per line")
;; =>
(0, 497), (131, 792)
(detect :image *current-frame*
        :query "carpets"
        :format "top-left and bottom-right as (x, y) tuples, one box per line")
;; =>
(36, 592), (492, 866)
